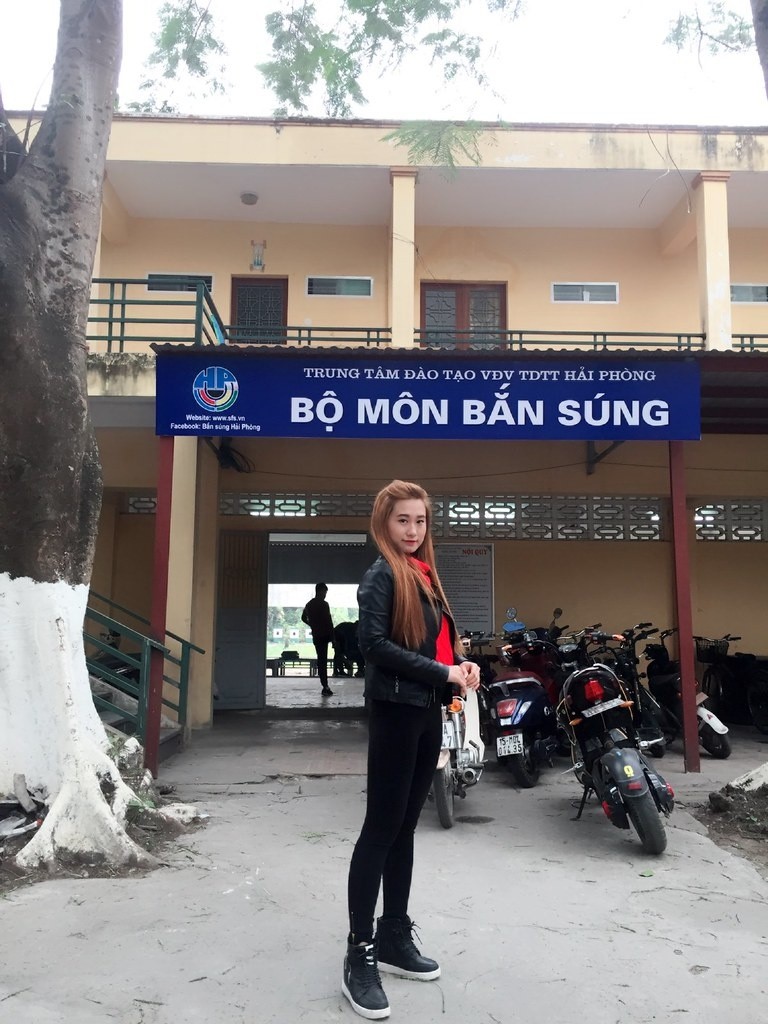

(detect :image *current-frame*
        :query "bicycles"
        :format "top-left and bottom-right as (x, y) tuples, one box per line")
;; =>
(588, 627), (680, 759)
(690, 633), (767, 736)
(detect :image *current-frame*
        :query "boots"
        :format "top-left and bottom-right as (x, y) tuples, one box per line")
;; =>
(372, 914), (440, 980)
(341, 931), (391, 1019)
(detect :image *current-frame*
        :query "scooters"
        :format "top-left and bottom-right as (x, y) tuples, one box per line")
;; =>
(636, 624), (732, 759)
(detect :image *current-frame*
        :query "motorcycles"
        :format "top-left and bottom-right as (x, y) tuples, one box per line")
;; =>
(425, 607), (675, 855)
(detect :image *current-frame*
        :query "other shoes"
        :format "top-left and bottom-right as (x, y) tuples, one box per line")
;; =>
(321, 688), (333, 696)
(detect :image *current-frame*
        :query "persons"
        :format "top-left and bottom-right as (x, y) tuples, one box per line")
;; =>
(341, 480), (481, 1020)
(302, 582), (364, 695)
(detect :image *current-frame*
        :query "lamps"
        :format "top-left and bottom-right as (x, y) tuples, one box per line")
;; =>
(240, 191), (259, 206)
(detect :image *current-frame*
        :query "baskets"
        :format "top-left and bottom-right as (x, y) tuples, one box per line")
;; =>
(696, 639), (729, 663)
